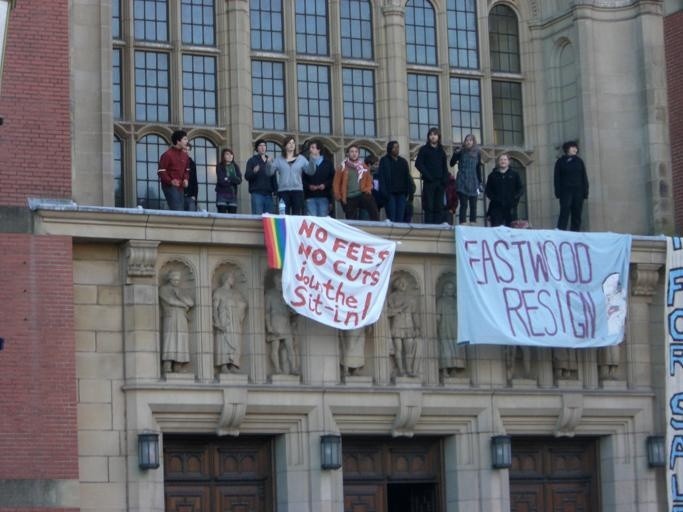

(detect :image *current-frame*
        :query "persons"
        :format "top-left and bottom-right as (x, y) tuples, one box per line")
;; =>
(387, 277), (420, 376)
(213, 148), (242, 213)
(484, 153), (526, 229)
(551, 140), (589, 231)
(551, 346), (578, 382)
(338, 326), (368, 376)
(210, 270), (248, 375)
(264, 273), (301, 375)
(597, 346), (619, 382)
(159, 270), (195, 373)
(180, 140), (197, 211)
(437, 283), (467, 378)
(155, 130), (191, 210)
(244, 126), (485, 227)
(505, 345), (536, 384)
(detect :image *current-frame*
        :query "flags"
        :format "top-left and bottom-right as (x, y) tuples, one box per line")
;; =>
(263, 213), (400, 332)
(452, 223), (632, 348)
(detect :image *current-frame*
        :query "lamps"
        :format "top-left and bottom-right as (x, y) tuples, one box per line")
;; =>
(491, 436), (512, 470)
(320, 434), (342, 470)
(646, 436), (666, 467)
(136, 434), (159, 469)
